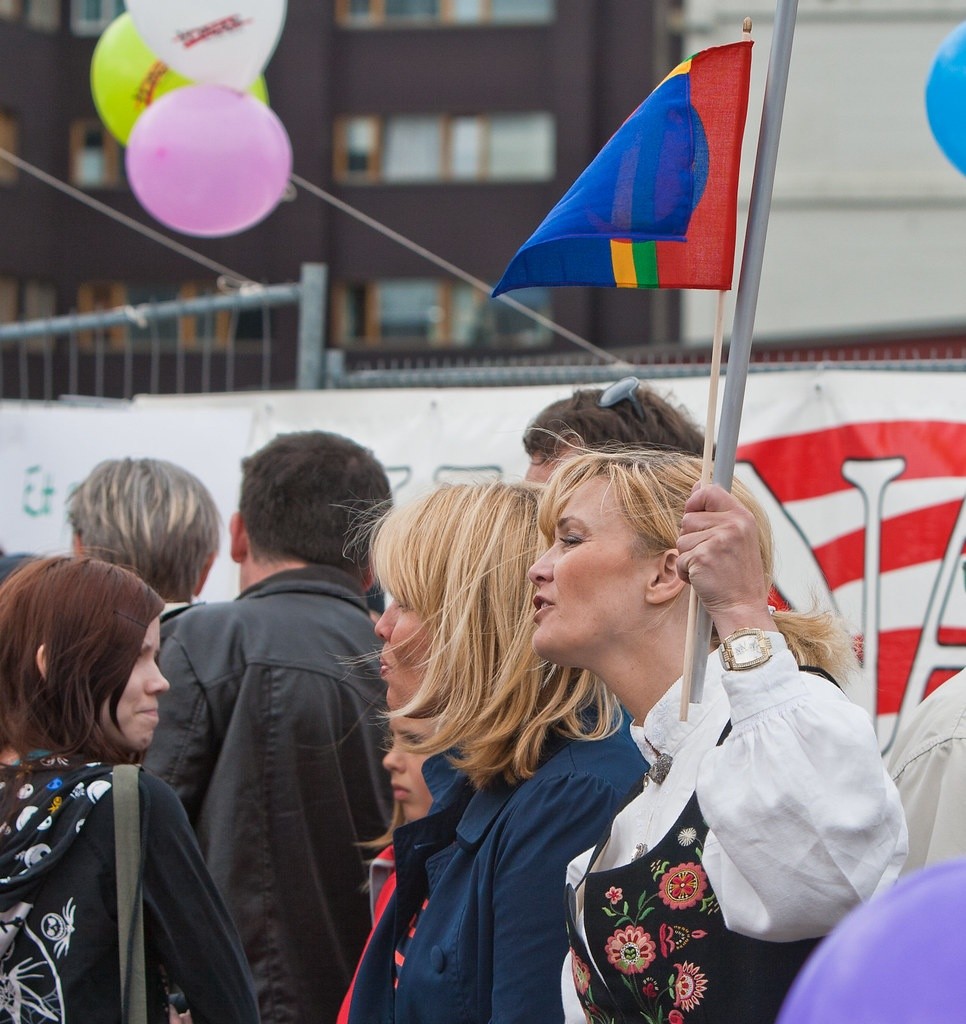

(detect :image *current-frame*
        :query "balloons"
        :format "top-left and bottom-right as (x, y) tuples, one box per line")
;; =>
(922, 17), (966, 184)
(118, 0), (293, 96)
(88, 12), (273, 152)
(769, 856), (965, 1024)
(122, 84), (294, 240)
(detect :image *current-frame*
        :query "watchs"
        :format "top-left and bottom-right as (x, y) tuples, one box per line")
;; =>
(717, 624), (791, 674)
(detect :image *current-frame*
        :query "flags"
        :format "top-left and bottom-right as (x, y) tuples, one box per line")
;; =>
(489, 38), (760, 323)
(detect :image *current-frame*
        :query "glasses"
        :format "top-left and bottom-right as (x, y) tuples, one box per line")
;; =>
(595, 375), (645, 423)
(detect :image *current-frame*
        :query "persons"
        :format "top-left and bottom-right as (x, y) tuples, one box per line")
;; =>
(60, 452), (234, 611)
(523, 445), (916, 1024)
(885, 643), (966, 891)
(330, 478), (655, 1024)
(134, 425), (407, 1024)
(513, 380), (723, 492)
(379, 692), (454, 836)
(0, 552), (270, 1024)
(364, 577), (384, 626)
